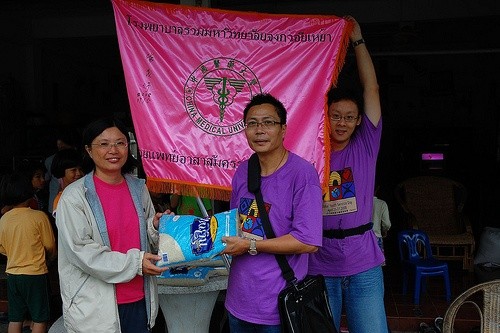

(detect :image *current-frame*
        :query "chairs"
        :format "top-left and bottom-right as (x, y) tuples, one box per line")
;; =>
(399, 230), (451, 305)
(442, 280), (500, 333)
(395, 176), (475, 271)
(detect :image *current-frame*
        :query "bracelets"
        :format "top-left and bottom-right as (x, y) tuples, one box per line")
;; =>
(353, 39), (365, 48)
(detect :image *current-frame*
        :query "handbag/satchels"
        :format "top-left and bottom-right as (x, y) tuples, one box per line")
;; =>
(278, 273), (337, 333)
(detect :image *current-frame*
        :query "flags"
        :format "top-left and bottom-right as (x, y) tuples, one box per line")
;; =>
(110, 0), (355, 201)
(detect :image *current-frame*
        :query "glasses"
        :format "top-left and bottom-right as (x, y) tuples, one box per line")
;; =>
(329, 113), (359, 123)
(90, 141), (129, 150)
(245, 120), (282, 128)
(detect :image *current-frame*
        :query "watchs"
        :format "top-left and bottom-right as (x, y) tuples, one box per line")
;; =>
(248, 239), (257, 256)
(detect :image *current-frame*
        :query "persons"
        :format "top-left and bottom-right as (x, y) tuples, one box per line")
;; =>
(372, 182), (391, 252)
(0, 150), (86, 333)
(219, 93), (323, 333)
(150, 188), (215, 219)
(308, 15), (387, 333)
(56, 118), (175, 333)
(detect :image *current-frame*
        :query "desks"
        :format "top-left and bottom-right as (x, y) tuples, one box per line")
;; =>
(158, 276), (229, 333)
